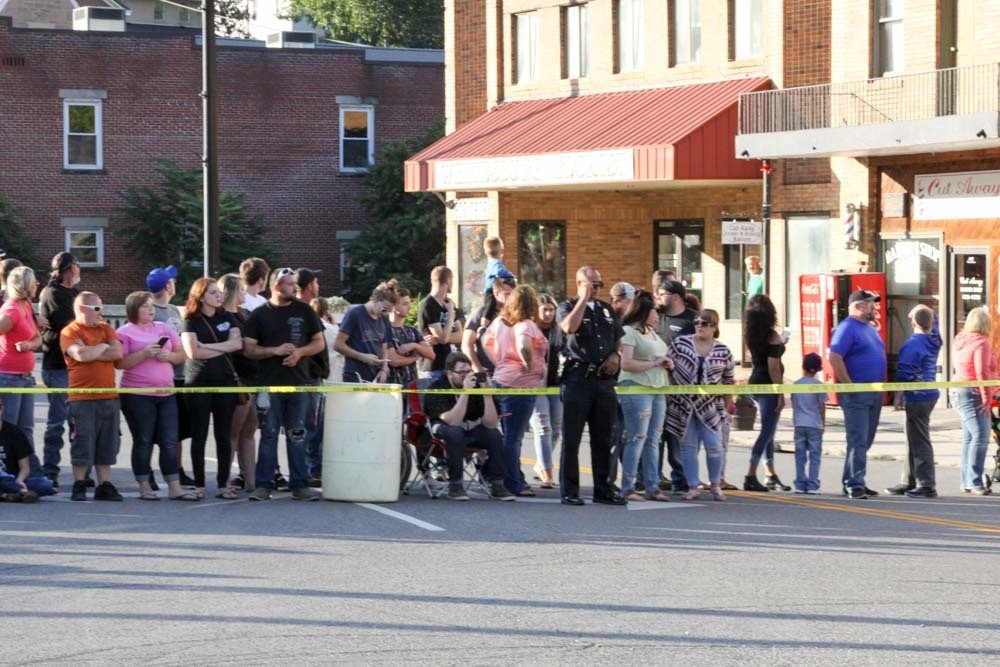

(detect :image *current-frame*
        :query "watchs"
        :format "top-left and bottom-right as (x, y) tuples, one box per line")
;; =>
(613, 350), (622, 357)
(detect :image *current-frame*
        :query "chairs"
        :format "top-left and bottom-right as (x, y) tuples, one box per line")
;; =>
(402, 378), (511, 498)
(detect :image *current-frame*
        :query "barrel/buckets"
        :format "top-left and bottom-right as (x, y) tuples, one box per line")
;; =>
(321, 381), (402, 501)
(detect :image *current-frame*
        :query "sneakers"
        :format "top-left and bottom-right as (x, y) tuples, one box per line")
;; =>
(905, 485), (936, 499)
(884, 482), (914, 495)
(842, 484), (877, 499)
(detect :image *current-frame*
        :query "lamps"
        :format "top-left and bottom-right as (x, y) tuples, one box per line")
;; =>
(843, 202), (862, 252)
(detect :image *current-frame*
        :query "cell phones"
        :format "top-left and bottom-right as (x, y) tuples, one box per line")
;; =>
(157, 336), (169, 348)
(472, 372), (487, 382)
(781, 331), (791, 344)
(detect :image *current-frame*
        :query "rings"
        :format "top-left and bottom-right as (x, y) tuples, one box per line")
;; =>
(429, 338), (431, 340)
(983, 408), (984, 409)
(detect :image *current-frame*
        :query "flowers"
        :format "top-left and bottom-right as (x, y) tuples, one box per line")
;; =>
(734, 394), (755, 408)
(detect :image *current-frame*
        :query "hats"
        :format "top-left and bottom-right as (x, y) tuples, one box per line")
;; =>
(146, 265), (178, 292)
(296, 267), (322, 288)
(804, 353), (822, 372)
(850, 291), (881, 306)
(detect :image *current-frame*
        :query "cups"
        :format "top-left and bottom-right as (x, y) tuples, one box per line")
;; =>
(780, 331), (791, 343)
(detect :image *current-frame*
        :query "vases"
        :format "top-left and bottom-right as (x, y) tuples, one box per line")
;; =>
(730, 406), (758, 430)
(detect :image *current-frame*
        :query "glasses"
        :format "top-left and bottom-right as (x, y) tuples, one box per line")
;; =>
(276, 268), (294, 285)
(79, 304), (103, 313)
(448, 368), (473, 376)
(588, 282), (603, 288)
(378, 300), (393, 314)
(693, 320), (710, 327)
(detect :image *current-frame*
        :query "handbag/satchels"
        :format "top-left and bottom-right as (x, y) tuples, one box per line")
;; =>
(235, 377), (249, 405)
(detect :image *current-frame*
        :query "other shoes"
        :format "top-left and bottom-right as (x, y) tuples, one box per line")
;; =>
(967, 484), (991, 495)
(0, 462), (818, 507)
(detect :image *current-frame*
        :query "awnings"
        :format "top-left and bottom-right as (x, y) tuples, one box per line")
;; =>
(406, 78), (773, 208)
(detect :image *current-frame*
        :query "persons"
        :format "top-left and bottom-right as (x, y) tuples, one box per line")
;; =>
(481, 285), (548, 498)
(610, 282), (635, 318)
(828, 291), (886, 499)
(655, 279), (701, 346)
(744, 295), (793, 491)
(477, 237), (516, 341)
(951, 307), (1000, 495)
(707, 402), (738, 490)
(461, 277), (516, 436)
(424, 351), (517, 501)
(791, 353), (829, 495)
(886, 304), (943, 499)
(417, 266), (463, 373)
(745, 256), (764, 297)
(651, 270), (674, 296)
(556, 267), (627, 504)
(615, 290), (669, 502)
(664, 309), (734, 502)
(0, 251), (436, 503)
(528, 294), (563, 486)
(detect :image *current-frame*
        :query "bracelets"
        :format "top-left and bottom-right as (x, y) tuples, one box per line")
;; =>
(381, 369), (389, 375)
(362, 354), (368, 363)
